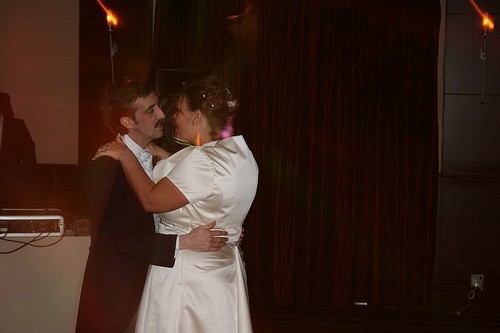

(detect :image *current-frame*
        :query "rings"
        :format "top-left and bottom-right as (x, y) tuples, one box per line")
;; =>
(100, 147), (105, 152)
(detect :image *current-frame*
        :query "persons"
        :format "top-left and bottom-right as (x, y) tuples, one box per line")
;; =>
(91, 76), (259, 333)
(75, 79), (245, 333)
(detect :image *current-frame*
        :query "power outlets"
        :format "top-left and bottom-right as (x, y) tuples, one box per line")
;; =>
(470, 274), (483, 288)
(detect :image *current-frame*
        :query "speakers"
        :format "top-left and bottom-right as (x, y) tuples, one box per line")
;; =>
(153, 66), (212, 154)
